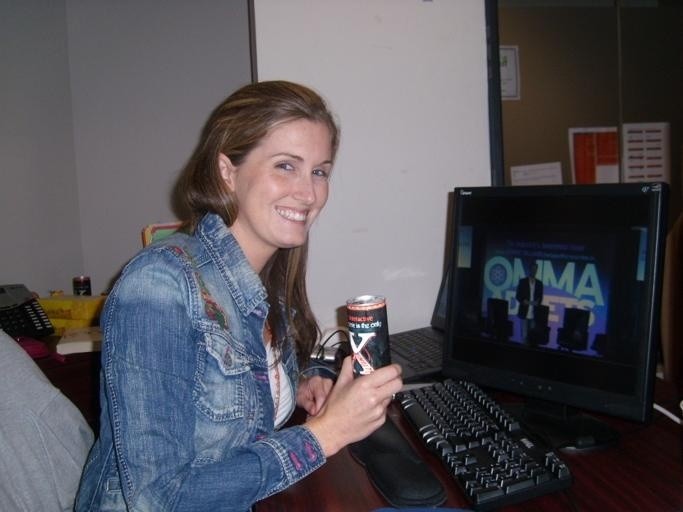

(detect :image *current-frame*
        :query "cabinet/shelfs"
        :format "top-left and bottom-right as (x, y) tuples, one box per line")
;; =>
(48, 339), (103, 432)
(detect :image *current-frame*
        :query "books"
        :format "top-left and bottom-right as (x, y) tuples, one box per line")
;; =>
(56, 324), (102, 356)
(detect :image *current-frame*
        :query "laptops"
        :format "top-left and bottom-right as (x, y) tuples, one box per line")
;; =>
(389, 261), (449, 383)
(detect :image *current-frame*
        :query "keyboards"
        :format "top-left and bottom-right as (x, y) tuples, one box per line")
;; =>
(396, 381), (573, 505)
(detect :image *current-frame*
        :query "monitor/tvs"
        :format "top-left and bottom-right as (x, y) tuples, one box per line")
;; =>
(441, 182), (667, 440)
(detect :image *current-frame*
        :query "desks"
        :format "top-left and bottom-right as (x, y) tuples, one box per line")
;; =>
(249, 381), (682, 511)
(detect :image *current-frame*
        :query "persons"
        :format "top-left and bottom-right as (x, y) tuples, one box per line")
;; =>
(70, 82), (405, 511)
(516, 263), (543, 345)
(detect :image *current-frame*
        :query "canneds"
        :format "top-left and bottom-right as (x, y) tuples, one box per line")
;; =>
(347, 295), (391, 379)
(72, 277), (91, 296)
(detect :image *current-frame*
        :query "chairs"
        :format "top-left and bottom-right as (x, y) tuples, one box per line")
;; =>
(0, 328), (97, 511)
